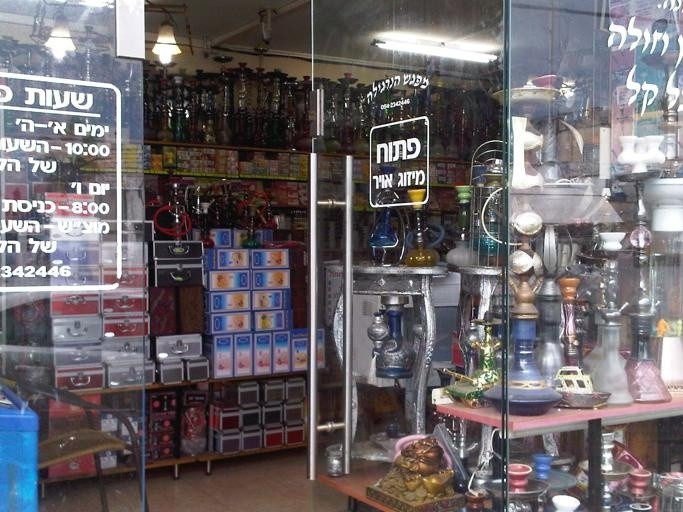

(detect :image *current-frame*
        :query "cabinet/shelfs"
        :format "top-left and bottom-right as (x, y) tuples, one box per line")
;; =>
(308, 386), (683, 511)
(1, 139), (493, 500)
